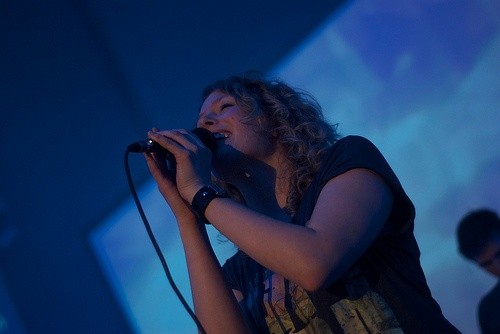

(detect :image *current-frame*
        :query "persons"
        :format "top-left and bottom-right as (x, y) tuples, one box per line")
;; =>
(141, 69), (463, 334)
(457, 206), (499, 334)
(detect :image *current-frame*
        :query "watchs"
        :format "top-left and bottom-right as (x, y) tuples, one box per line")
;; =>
(189, 182), (231, 225)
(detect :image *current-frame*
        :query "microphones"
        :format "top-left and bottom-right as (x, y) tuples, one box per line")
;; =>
(129, 127), (219, 167)
